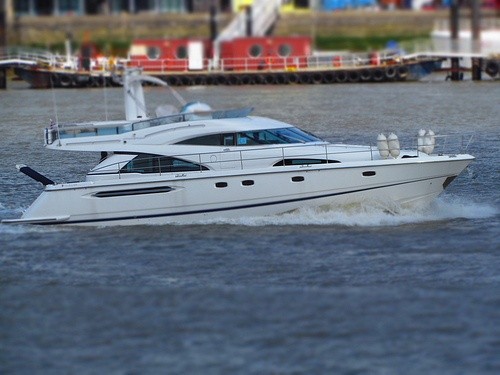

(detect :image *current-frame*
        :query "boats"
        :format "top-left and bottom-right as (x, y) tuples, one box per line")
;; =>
(0, 62), (475, 224)
(1, 6), (496, 85)
